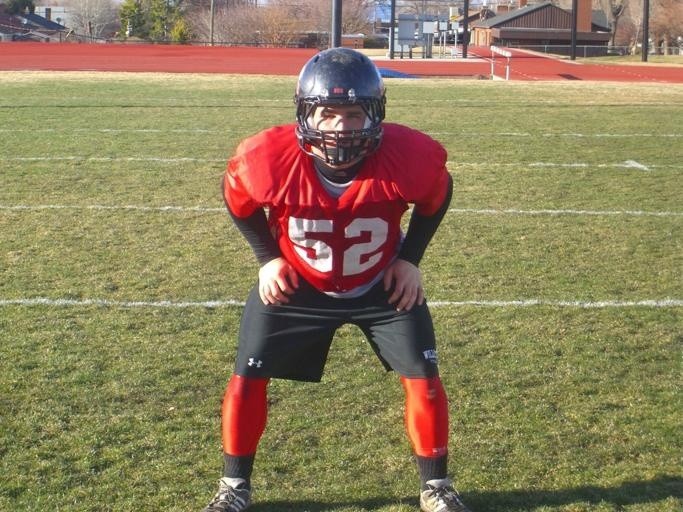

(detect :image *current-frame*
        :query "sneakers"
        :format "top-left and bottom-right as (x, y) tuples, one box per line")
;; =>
(419, 478), (467, 512)
(203, 478), (252, 511)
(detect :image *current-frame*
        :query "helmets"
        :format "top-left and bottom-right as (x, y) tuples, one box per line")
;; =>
(294, 48), (386, 165)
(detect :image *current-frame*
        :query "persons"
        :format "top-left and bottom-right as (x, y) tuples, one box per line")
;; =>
(201, 47), (471, 512)
